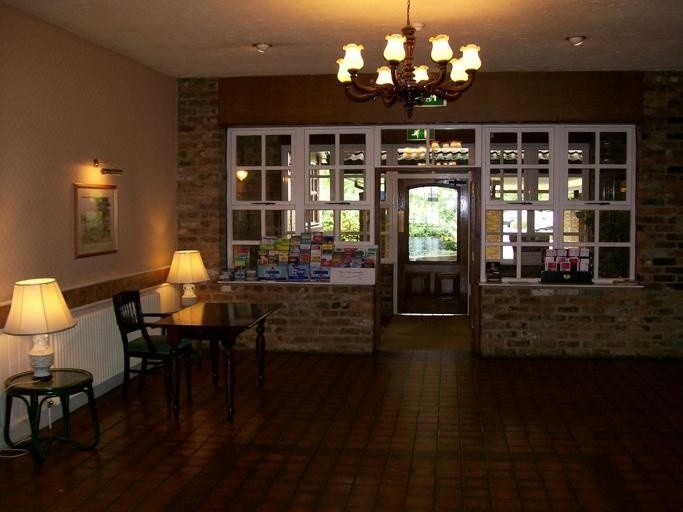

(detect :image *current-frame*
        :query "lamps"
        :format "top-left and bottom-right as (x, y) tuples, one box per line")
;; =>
(336, 0), (482, 119)
(4, 277), (77, 381)
(399, 137), (464, 171)
(568, 36), (584, 46)
(236, 154), (249, 181)
(256, 44), (269, 54)
(166, 250), (210, 307)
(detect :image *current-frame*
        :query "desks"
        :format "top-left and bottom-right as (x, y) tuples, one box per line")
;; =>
(152, 302), (287, 425)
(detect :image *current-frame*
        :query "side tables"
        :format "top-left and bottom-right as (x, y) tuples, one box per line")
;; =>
(6, 368), (100, 463)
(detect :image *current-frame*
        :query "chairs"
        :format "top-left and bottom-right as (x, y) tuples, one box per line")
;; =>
(112, 290), (192, 416)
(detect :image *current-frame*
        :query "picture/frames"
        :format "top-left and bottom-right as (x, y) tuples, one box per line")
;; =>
(72, 184), (122, 259)
